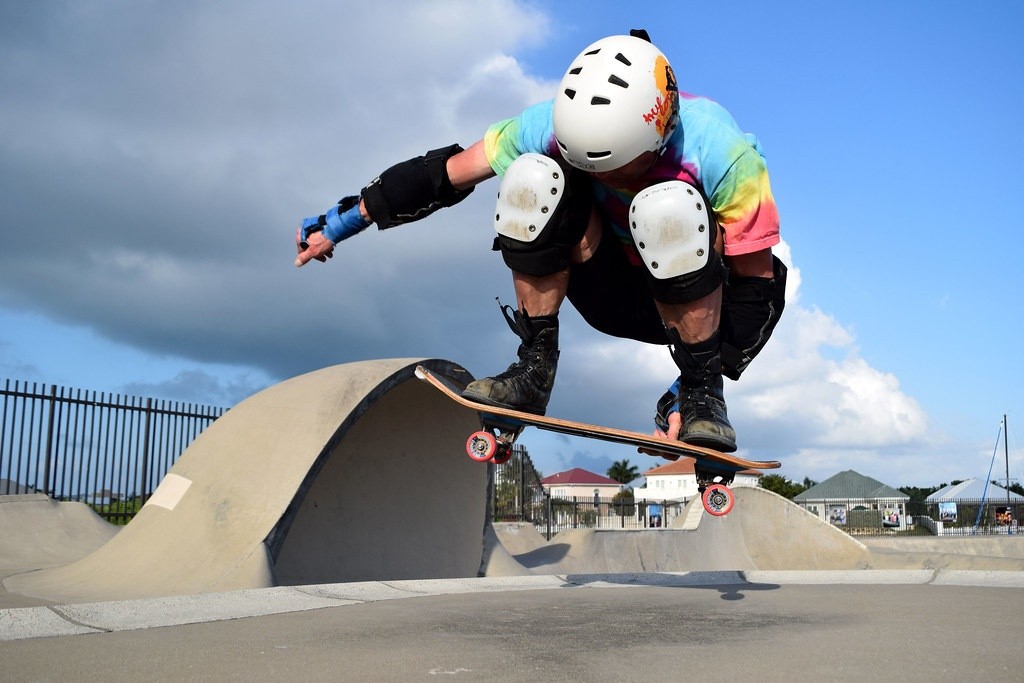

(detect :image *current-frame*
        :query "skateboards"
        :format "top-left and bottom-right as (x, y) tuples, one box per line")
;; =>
(414, 362), (782, 516)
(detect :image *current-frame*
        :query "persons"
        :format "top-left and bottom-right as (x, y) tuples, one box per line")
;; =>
(831, 508), (845, 522)
(887, 510), (899, 522)
(295, 30), (788, 461)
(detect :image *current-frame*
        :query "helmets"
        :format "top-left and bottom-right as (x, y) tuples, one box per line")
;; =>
(551, 30), (682, 188)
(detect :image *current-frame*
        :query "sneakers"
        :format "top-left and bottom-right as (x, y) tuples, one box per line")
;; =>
(462, 298), (561, 415)
(674, 344), (738, 452)
